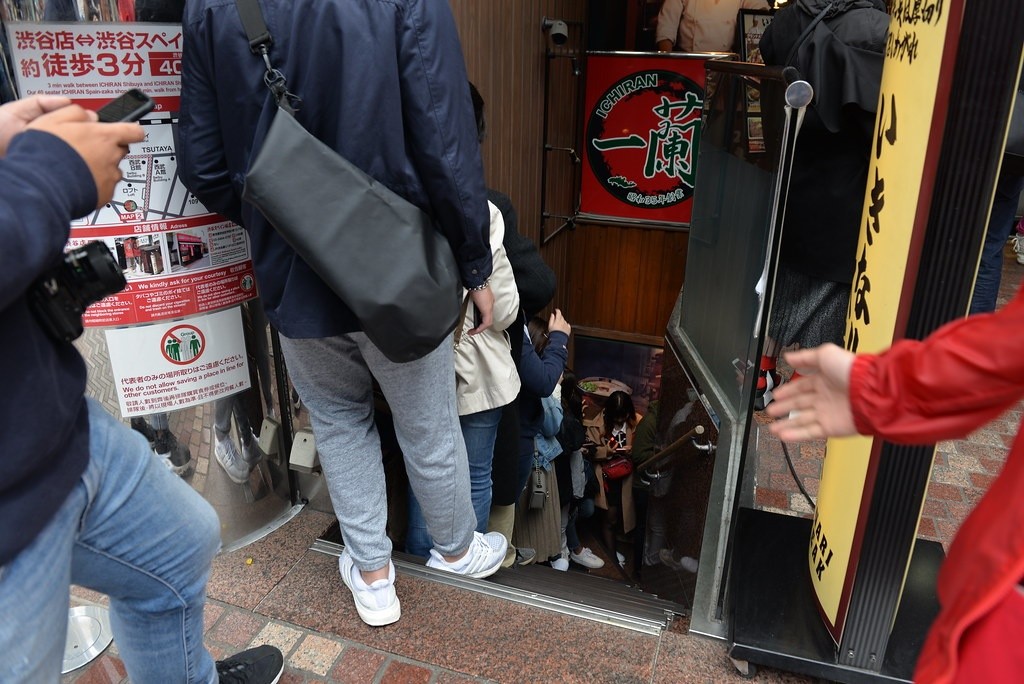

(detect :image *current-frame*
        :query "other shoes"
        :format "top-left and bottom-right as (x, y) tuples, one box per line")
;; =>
(550, 558), (569, 571)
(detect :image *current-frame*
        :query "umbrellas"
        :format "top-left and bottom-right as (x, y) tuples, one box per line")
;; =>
(577, 376), (633, 397)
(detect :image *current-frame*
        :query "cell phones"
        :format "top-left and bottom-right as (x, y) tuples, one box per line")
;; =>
(616, 448), (626, 452)
(94, 89), (154, 125)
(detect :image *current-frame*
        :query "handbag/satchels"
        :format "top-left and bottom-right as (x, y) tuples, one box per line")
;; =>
(234, 0), (463, 364)
(570, 451), (585, 499)
(525, 470), (550, 516)
(601, 456), (633, 479)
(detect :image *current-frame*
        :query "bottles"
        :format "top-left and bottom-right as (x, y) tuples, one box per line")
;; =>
(609, 436), (617, 447)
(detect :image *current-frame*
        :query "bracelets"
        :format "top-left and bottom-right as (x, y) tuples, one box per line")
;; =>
(468, 282), (488, 291)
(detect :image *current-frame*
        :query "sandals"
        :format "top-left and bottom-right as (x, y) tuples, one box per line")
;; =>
(753, 369), (785, 411)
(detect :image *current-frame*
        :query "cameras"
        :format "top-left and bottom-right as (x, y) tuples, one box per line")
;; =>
(28, 241), (129, 349)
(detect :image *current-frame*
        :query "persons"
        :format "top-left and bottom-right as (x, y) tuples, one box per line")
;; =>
(0, 93), (285, 684)
(174, 0), (508, 626)
(655, 0), (1024, 410)
(766, 284), (1024, 684)
(415, 78), (720, 585)
(129, 296), (278, 482)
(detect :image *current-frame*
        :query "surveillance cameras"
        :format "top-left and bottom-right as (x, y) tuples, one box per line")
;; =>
(550, 20), (568, 45)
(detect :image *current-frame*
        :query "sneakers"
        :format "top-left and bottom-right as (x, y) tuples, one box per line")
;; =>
(339, 547), (401, 626)
(515, 548), (536, 566)
(571, 547), (604, 569)
(215, 644), (284, 684)
(153, 429), (191, 475)
(680, 556), (698, 574)
(213, 425), (249, 484)
(241, 435), (261, 473)
(1011, 233), (1024, 265)
(659, 549), (682, 571)
(131, 417), (157, 448)
(425, 531), (507, 578)
